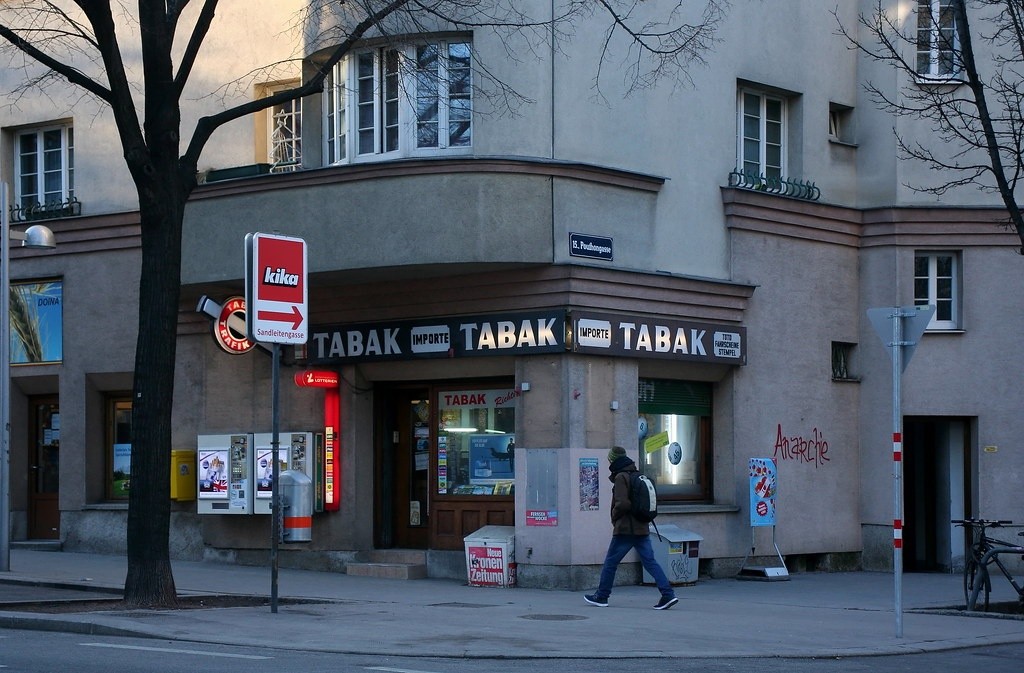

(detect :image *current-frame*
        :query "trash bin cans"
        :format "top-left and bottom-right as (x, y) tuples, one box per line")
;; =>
(643, 524), (705, 586)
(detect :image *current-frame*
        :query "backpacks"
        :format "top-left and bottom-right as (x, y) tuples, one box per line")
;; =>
(612, 471), (657, 523)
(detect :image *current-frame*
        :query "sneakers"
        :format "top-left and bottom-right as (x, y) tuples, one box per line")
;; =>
(584, 595), (609, 606)
(652, 595), (678, 609)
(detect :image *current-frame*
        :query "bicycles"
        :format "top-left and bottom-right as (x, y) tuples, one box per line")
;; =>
(950, 519), (1024, 614)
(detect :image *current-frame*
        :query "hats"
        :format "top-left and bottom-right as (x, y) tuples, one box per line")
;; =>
(608, 446), (626, 463)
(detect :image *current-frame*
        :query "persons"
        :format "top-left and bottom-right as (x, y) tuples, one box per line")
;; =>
(584, 446), (679, 610)
(507, 438), (515, 472)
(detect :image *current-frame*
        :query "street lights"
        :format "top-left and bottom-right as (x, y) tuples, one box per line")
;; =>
(0, 180), (59, 571)
